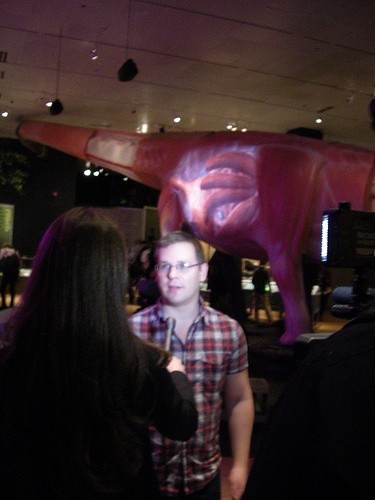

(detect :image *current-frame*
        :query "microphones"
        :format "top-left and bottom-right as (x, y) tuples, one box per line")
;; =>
(164, 317), (175, 352)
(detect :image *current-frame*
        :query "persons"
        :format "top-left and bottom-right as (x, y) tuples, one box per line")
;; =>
(315, 262), (333, 321)
(127, 230), (254, 500)
(350, 259), (369, 314)
(1, 207), (200, 500)
(251, 257), (274, 323)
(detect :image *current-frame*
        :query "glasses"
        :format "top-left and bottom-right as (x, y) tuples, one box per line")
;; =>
(155, 261), (205, 272)
